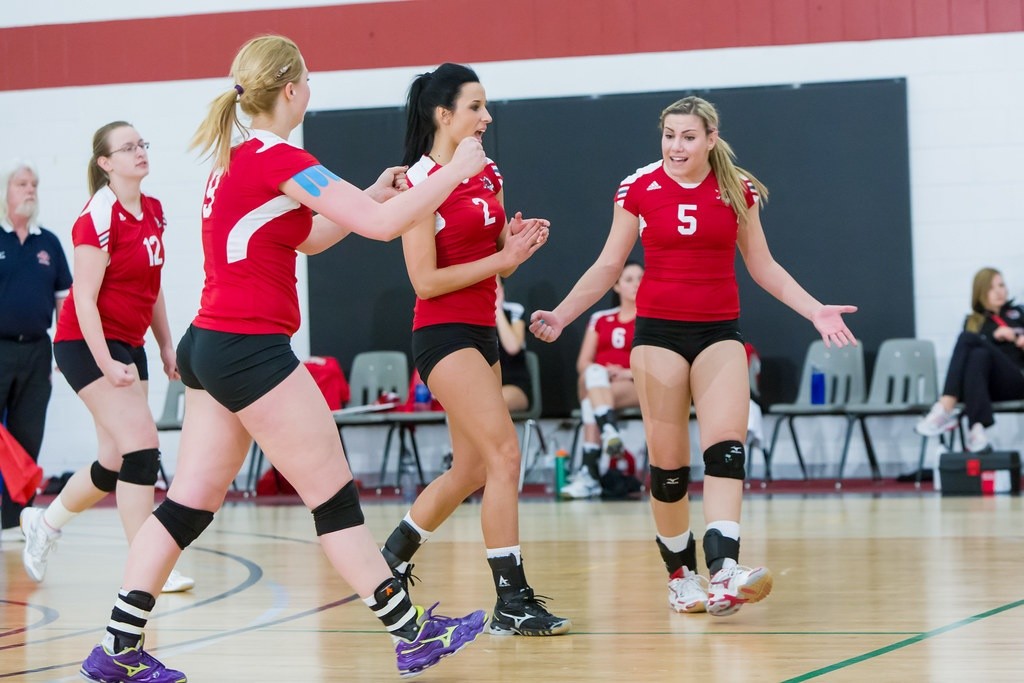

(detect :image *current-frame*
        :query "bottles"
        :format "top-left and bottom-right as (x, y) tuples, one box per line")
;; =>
(811, 365), (825, 405)
(401, 451), (417, 499)
(555, 445), (571, 499)
(414, 376), (431, 412)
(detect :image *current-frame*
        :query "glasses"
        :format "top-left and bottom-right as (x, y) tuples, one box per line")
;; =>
(109, 142), (149, 154)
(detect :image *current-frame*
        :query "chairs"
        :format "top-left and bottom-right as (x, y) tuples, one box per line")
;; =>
(745, 335), (1024, 489)
(570, 407), (698, 496)
(155, 350), (550, 498)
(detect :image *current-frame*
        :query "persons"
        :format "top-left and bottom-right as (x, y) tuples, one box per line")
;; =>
(79, 34), (490, 683)
(529, 97), (858, 617)
(19, 122), (194, 593)
(559, 261), (644, 499)
(379, 63), (572, 636)
(915, 269), (1024, 453)
(0, 162), (68, 542)
(495, 273), (534, 412)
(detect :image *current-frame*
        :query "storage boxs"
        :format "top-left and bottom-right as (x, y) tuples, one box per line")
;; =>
(937, 449), (1021, 495)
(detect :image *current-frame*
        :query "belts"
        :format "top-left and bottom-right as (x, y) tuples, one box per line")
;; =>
(0, 336), (47, 344)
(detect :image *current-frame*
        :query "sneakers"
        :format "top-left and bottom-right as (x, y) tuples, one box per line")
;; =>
(668, 565), (709, 613)
(965, 427), (986, 452)
(162, 571), (194, 592)
(19, 507), (62, 582)
(79, 632), (187, 683)
(606, 437), (623, 459)
(915, 402), (961, 436)
(488, 587), (571, 636)
(706, 558), (773, 616)
(393, 601), (491, 679)
(560, 466), (602, 498)
(381, 547), (422, 597)
